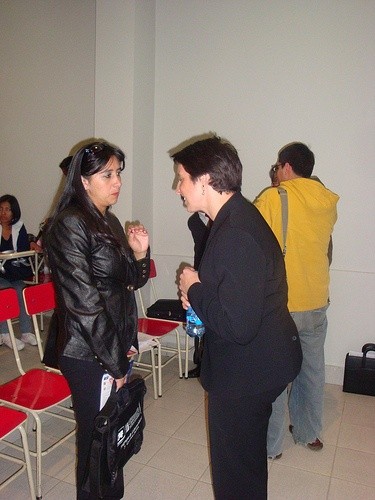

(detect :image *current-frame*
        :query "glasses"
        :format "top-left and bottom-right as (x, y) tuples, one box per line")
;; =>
(85, 139), (110, 156)
(272, 162), (286, 171)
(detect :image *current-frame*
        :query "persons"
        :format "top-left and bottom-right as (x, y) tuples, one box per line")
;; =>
(40, 142), (150, 500)
(252, 142), (340, 462)
(188, 211), (214, 378)
(0, 194), (43, 350)
(173, 135), (303, 500)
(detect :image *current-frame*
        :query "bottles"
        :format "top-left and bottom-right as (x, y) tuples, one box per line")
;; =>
(185, 307), (207, 337)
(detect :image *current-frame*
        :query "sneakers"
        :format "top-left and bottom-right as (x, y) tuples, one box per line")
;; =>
(21, 333), (44, 346)
(0, 334), (25, 352)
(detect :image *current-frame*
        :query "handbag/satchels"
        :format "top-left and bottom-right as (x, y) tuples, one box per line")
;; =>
(342, 344), (375, 397)
(91, 378), (147, 484)
(146, 299), (186, 323)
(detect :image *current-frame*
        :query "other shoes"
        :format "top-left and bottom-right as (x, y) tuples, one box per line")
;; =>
(182, 368), (199, 378)
(289, 425), (323, 450)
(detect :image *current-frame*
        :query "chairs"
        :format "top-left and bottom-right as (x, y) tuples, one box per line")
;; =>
(0, 247), (78, 500)
(126, 259), (202, 400)
